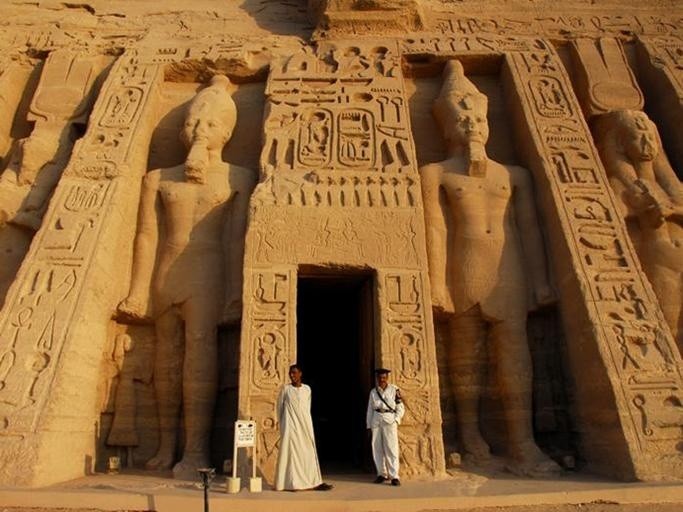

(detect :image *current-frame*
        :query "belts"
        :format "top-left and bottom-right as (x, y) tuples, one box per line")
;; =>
(376, 409), (396, 412)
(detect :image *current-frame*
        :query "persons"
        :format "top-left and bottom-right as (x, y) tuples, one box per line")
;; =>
(275, 364), (334, 492)
(609, 108), (683, 361)
(116, 68), (243, 478)
(365, 369), (406, 487)
(418, 59), (563, 478)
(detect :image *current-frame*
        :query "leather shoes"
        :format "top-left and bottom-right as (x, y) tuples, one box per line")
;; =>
(314, 482), (334, 490)
(373, 475), (401, 486)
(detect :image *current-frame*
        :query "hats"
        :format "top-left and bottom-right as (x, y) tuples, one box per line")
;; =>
(376, 368), (391, 373)
